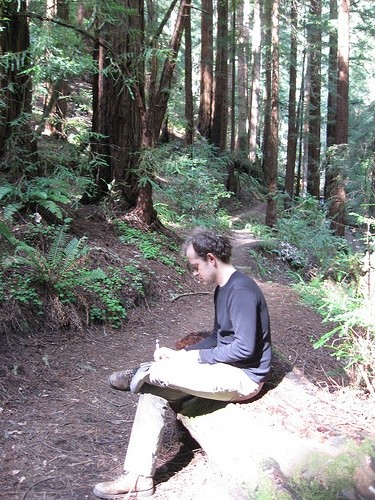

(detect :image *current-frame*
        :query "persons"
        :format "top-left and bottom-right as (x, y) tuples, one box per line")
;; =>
(92, 231), (271, 499)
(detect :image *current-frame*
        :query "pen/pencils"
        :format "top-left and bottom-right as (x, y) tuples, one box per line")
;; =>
(155, 339), (159, 349)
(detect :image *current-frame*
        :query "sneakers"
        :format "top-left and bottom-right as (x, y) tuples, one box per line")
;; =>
(108, 365), (143, 391)
(93, 474), (155, 499)
